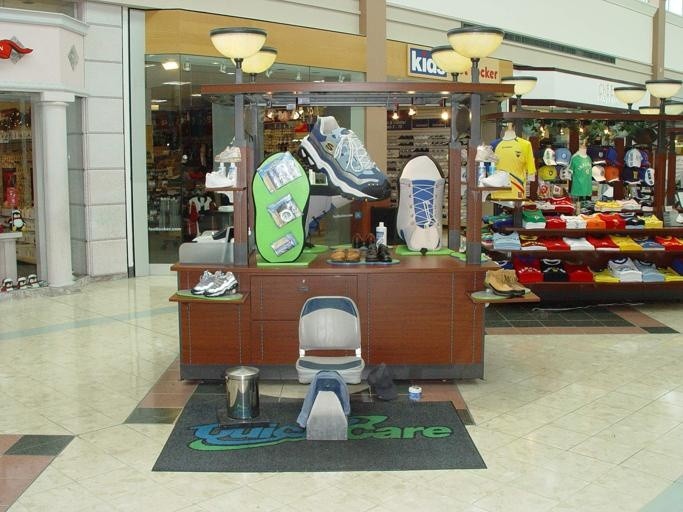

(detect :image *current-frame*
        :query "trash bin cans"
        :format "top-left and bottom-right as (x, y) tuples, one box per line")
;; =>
(224, 366), (260, 420)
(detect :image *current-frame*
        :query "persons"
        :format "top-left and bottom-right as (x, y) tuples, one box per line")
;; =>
(166, 159), (200, 242)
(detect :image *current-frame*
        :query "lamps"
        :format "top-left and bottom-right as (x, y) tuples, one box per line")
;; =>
(431, 46), (475, 76)
(219, 60), (227, 75)
(210, 27), (268, 69)
(665, 104), (682, 113)
(614, 86), (645, 106)
(641, 106), (660, 116)
(445, 26), (504, 67)
(264, 68), (274, 77)
(230, 46), (278, 76)
(295, 68), (302, 81)
(182, 56), (192, 72)
(646, 80), (681, 103)
(337, 73), (346, 82)
(501, 75), (538, 98)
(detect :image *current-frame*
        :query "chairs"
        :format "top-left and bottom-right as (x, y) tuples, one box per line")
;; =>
(295, 295), (365, 385)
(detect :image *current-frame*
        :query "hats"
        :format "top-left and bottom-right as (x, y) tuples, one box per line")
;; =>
(640, 151), (651, 165)
(543, 148), (557, 166)
(556, 148), (571, 165)
(622, 167), (641, 184)
(625, 149), (643, 168)
(587, 145), (607, 165)
(592, 165), (607, 181)
(560, 167), (573, 180)
(605, 166), (620, 182)
(640, 167), (654, 186)
(539, 166), (557, 180)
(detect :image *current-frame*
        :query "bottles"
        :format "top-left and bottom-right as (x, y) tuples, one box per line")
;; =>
(376, 222), (387, 246)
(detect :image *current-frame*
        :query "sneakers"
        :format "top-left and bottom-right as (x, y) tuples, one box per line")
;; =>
(347, 247), (360, 262)
(331, 248), (345, 262)
(365, 244), (378, 261)
(475, 146), (499, 162)
(215, 146), (241, 163)
(503, 270), (525, 296)
(191, 271), (224, 294)
(204, 272), (238, 297)
(481, 172), (510, 187)
(205, 172), (233, 188)
(484, 269), (513, 296)
(377, 244), (392, 262)
(297, 116), (391, 202)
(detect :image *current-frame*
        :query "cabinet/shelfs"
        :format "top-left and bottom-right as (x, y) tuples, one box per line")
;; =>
(170, 82), (539, 381)
(479, 111), (683, 305)
(387, 131), (467, 229)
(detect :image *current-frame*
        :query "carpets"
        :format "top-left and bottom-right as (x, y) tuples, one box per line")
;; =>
(150, 392), (487, 472)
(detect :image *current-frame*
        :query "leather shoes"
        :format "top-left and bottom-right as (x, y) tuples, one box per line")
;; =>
(353, 233), (363, 248)
(366, 233), (375, 247)
